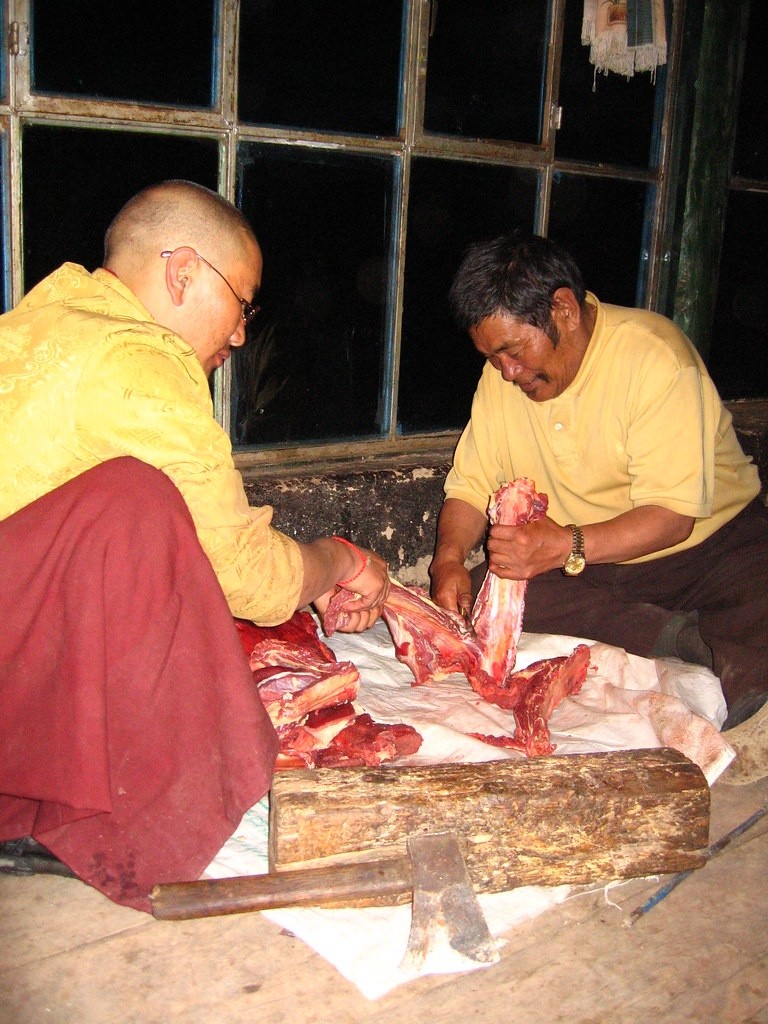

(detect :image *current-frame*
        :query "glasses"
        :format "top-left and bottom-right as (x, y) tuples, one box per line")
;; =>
(160, 247), (261, 326)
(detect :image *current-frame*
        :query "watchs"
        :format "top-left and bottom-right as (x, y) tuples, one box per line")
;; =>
(561, 524), (586, 577)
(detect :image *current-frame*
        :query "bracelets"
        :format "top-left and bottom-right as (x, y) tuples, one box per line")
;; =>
(329, 536), (372, 587)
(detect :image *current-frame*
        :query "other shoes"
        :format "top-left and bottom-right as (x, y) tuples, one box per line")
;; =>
(0, 836), (102, 883)
(715, 688), (768, 786)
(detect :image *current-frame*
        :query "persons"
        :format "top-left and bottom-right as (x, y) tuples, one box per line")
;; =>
(428, 231), (768, 733)
(0, 179), (391, 915)
